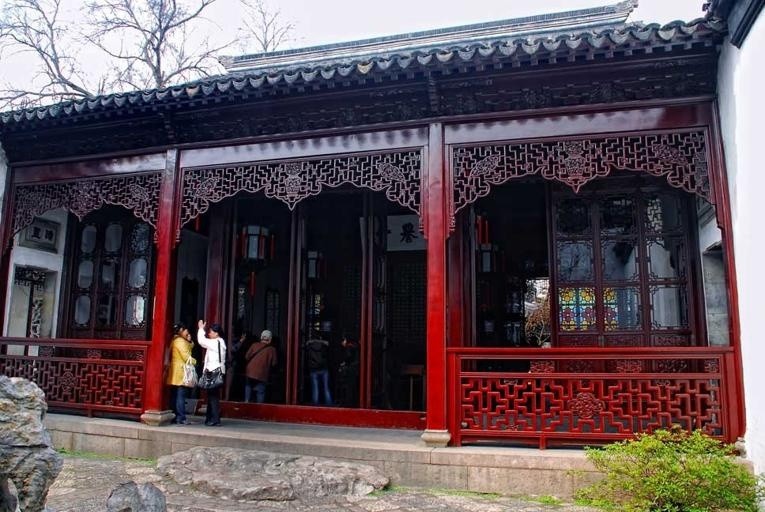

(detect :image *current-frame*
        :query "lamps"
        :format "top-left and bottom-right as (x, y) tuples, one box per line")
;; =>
(308, 250), (326, 281)
(235, 216), (277, 296)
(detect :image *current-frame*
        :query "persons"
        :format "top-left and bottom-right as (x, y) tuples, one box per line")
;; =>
(196, 318), (227, 426)
(166, 321), (198, 424)
(227, 323), (395, 411)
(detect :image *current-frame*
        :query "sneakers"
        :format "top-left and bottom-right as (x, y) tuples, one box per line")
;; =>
(177, 421), (191, 425)
(205, 421), (220, 426)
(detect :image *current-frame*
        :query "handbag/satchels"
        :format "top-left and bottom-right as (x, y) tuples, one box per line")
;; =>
(236, 358), (247, 377)
(198, 367), (223, 388)
(164, 347), (171, 366)
(182, 364), (198, 385)
